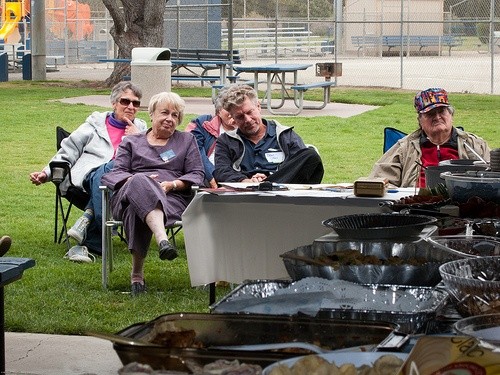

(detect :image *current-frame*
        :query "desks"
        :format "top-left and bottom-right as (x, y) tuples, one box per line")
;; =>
(481, 36), (500, 53)
(100, 58), (230, 87)
(230, 62), (313, 115)
(181, 182), (423, 309)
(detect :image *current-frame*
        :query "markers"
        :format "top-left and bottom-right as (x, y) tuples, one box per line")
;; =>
(386, 190), (399, 193)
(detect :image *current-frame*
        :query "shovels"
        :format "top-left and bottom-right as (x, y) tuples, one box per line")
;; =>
(79, 330), (374, 354)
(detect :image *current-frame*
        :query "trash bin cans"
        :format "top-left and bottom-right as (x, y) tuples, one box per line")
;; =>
(493, 31), (500, 53)
(99, 29), (110, 41)
(130, 48), (172, 110)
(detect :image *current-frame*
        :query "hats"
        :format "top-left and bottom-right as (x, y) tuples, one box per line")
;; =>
(414, 88), (450, 114)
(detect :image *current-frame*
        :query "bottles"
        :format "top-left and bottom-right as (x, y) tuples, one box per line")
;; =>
(54, 153), (64, 183)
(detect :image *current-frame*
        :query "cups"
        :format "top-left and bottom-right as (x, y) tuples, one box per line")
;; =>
(489, 148), (500, 172)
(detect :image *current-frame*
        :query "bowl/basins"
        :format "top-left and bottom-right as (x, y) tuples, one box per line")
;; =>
(439, 255), (500, 318)
(452, 313), (500, 346)
(433, 236), (499, 263)
(421, 158), (500, 195)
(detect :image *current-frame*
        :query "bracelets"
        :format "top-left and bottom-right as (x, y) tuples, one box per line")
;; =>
(172, 180), (177, 191)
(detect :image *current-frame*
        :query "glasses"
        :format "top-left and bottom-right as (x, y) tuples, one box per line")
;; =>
(120, 98), (140, 107)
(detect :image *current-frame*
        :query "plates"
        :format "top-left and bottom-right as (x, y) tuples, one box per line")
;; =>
(107, 210), (499, 375)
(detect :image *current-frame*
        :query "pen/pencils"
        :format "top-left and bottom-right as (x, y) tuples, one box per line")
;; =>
(320, 188), (341, 192)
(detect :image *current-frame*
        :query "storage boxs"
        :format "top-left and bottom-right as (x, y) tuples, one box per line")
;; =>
(354, 176), (389, 198)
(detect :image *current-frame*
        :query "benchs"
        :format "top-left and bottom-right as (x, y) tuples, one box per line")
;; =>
(46, 54), (63, 69)
(353, 33), (463, 53)
(478, 43), (500, 47)
(120, 25), (338, 92)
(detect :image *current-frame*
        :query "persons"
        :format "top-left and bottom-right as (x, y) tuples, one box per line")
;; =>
(29, 81), (324, 294)
(373, 87), (492, 188)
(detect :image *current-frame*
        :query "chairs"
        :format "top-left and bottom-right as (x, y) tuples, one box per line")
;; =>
(383, 124), (469, 152)
(49, 126), (182, 288)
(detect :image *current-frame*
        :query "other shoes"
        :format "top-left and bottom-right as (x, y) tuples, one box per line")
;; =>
(63, 244), (94, 264)
(67, 216), (90, 243)
(0, 235), (12, 258)
(130, 278), (146, 298)
(158, 240), (178, 260)
